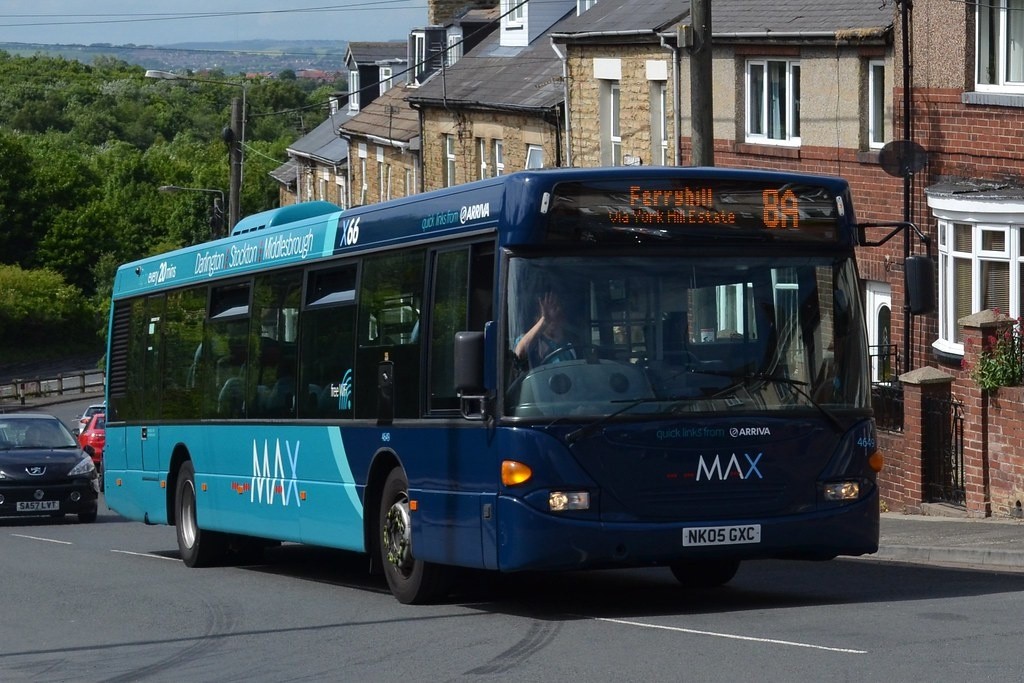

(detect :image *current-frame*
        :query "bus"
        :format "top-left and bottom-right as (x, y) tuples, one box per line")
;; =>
(102, 166), (938, 604)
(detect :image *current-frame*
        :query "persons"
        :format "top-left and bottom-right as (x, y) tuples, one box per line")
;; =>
(22, 428), (40, 445)
(512, 291), (583, 381)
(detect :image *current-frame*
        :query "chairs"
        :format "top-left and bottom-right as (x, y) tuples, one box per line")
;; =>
(267, 377), (295, 417)
(214, 377), (244, 417)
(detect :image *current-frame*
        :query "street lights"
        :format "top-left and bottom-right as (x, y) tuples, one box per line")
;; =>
(144, 68), (252, 239)
(156, 184), (231, 240)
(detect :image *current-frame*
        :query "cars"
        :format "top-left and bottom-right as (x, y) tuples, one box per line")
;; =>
(71, 411), (105, 471)
(0, 413), (100, 525)
(75, 405), (106, 438)
